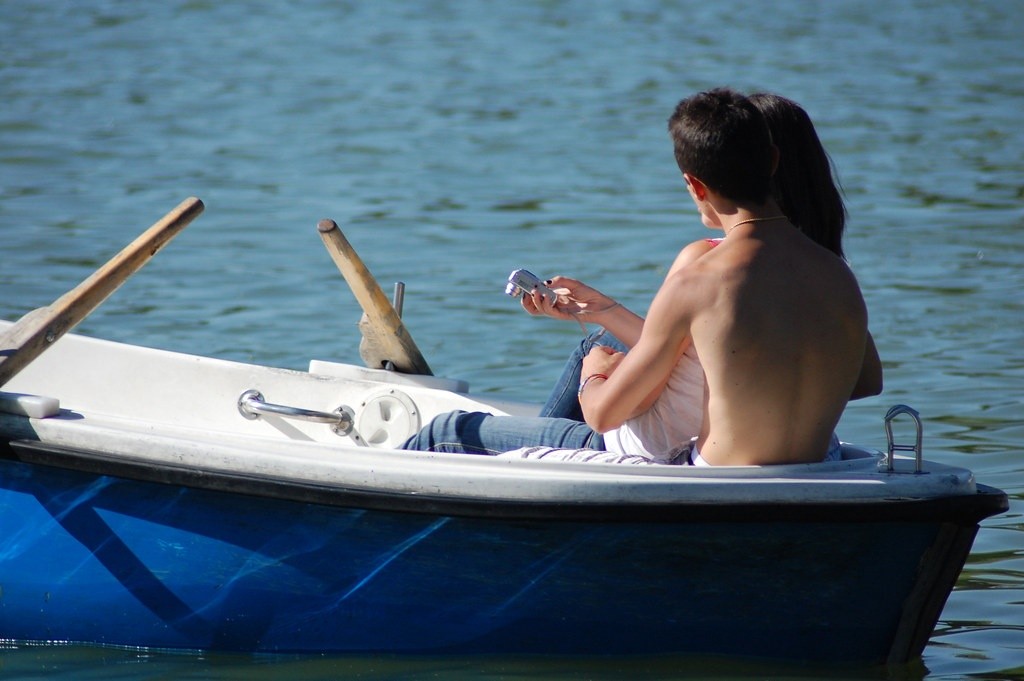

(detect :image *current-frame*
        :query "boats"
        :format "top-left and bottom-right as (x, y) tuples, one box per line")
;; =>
(0, 193), (1005, 663)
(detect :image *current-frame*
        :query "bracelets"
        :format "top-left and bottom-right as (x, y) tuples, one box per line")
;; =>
(578, 374), (608, 403)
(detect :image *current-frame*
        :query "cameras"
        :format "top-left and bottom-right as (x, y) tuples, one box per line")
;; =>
(505, 269), (559, 307)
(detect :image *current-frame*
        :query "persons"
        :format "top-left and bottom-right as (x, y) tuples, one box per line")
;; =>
(390, 93), (848, 457)
(494, 87), (884, 467)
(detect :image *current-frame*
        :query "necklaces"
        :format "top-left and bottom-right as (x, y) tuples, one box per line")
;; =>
(729, 216), (811, 220)
